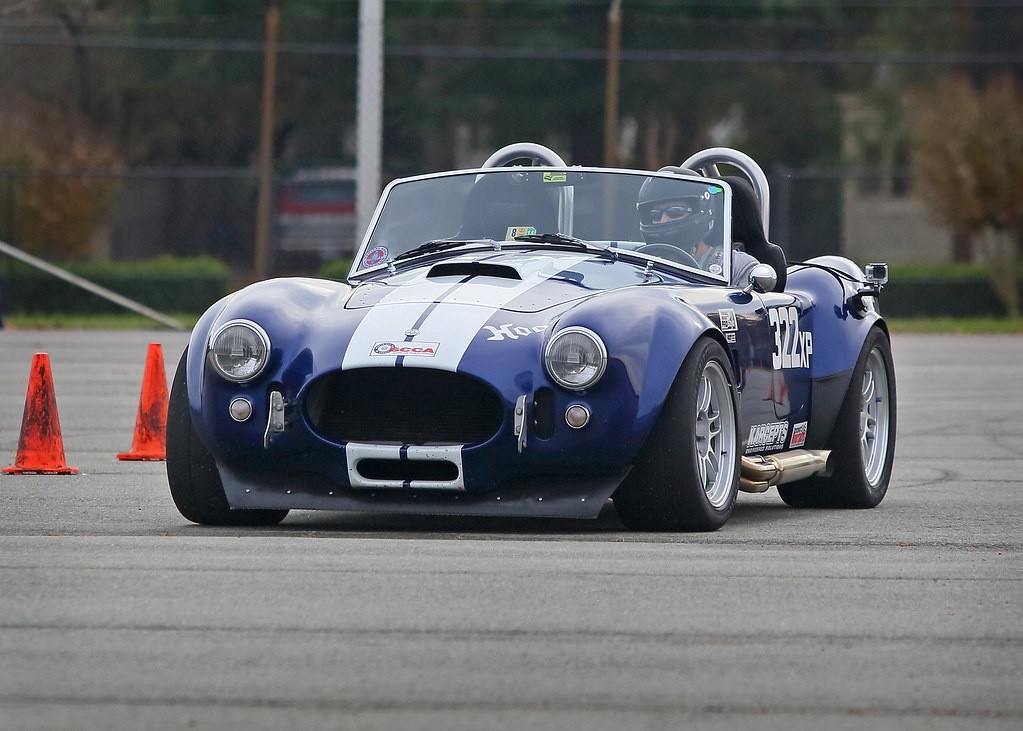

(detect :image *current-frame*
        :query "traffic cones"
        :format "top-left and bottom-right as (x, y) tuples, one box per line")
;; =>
(117, 344), (169, 462)
(4, 352), (78, 474)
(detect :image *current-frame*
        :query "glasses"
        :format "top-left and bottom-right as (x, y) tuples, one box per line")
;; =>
(647, 206), (695, 221)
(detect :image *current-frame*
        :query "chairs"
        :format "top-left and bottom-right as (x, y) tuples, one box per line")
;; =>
(680, 146), (788, 294)
(446, 142), (575, 242)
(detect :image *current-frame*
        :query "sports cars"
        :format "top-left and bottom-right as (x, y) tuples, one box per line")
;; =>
(165, 141), (898, 532)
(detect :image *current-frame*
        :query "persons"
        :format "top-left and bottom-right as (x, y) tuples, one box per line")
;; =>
(636, 166), (761, 291)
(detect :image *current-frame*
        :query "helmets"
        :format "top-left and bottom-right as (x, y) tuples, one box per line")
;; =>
(637, 166), (716, 250)
